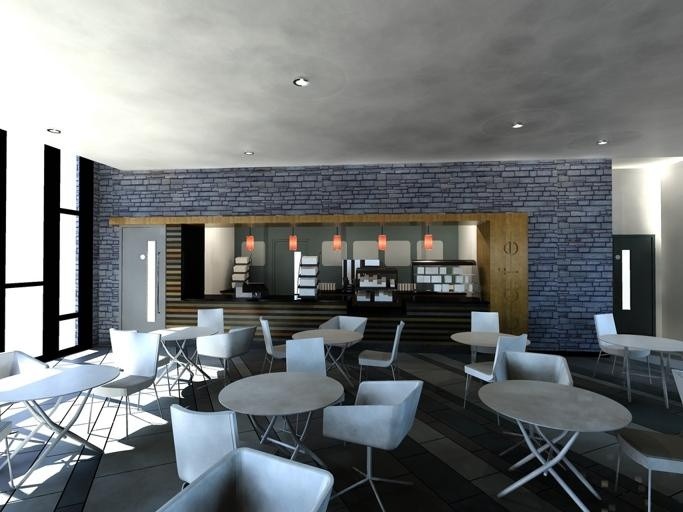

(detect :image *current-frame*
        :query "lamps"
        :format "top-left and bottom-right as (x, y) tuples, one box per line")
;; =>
(288, 223), (297, 250)
(376, 222), (386, 251)
(245, 223), (254, 251)
(332, 222), (341, 252)
(423, 222), (432, 250)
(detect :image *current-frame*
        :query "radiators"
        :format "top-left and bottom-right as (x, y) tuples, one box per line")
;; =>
(317, 283), (335, 290)
(398, 283), (413, 290)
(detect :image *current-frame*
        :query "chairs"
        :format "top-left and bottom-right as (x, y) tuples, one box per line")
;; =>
(277, 338), (326, 437)
(86, 332), (163, 440)
(319, 316), (367, 334)
(594, 313), (652, 387)
(196, 308), (223, 329)
(196, 326), (256, 389)
(614, 427), (683, 512)
(106, 327), (172, 408)
(0, 350), (49, 378)
(155, 447), (335, 512)
(258, 315), (286, 372)
(169, 403), (278, 491)
(471, 312), (499, 333)
(323, 381), (423, 512)
(463, 333), (528, 425)
(493, 351), (574, 477)
(358, 321), (404, 380)
